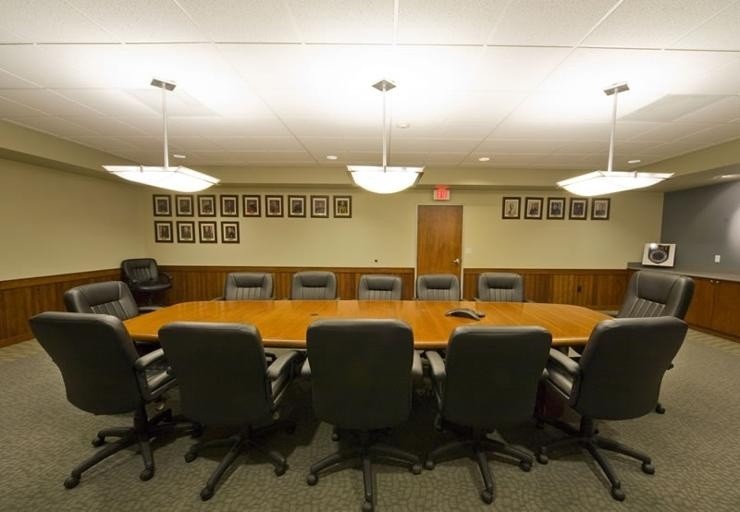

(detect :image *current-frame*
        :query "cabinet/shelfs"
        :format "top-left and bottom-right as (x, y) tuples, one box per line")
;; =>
(681, 275), (740, 339)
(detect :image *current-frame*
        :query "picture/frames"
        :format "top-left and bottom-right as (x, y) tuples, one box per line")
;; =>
(591, 198), (610, 220)
(524, 196), (543, 220)
(546, 197), (565, 220)
(569, 197), (588, 220)
(149, 191), (357, 244)
(502, 197), (521, 219)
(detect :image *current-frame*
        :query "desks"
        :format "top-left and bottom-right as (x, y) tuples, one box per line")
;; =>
(108, 299), (619, 348)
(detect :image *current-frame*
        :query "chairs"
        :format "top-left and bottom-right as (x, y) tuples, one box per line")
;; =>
(422, 328), (555, 505)
(303, 318), (433, 511)
(156, 322), (308, 502)
(209, 273), (274, 302)
(122, 258), (176, 305)
(416, 276), (462, 303)
(26, 312), (165, 489)
(473, 271), (536, 302)
(291, 270), (338, 301)
(546, 317), (688, 506)
(613, 266), (693, 415)
(64, 281), (163, 357)
(357, 274), (404, 300)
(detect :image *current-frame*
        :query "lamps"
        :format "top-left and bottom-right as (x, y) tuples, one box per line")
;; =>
(347, 75), (427, 196)
(557, 83), (676, 202)
(101, 78), (223, 198)
